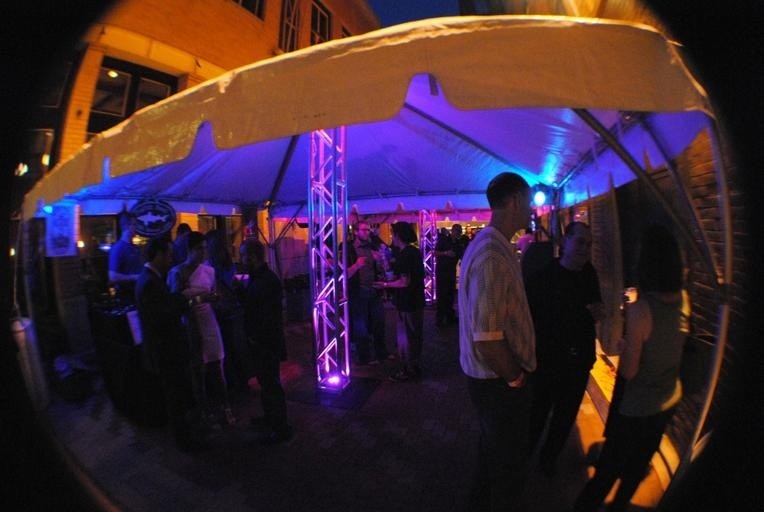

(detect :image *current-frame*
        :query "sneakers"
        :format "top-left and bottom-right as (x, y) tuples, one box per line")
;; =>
(385, 366), (417, 383)
(225, 408), (235, 424)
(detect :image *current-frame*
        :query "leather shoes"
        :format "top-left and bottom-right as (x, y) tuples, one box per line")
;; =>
(251, 415), (288, 425)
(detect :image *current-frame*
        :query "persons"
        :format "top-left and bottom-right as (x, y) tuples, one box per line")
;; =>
(339, 221), (425, 381)
(107, 212), (288, 445)
(435, 171), (603, 509)
(578, 227), (691, 510)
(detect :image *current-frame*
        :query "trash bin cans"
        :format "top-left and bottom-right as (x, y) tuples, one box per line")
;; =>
(285, 274), (312, 322)
(13, 317), (51, 410)
